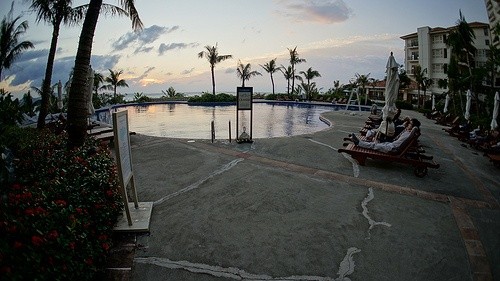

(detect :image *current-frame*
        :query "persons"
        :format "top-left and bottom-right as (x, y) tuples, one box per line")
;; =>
(340, 105), (421, 151)
(428, 101), (500, 161)
(238, 125), (250, 142)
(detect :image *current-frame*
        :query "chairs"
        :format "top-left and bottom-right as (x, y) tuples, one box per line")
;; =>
(311, 96), (348, 103)
(87, 121), (136, 146)
(337, 109), (500, 171)
